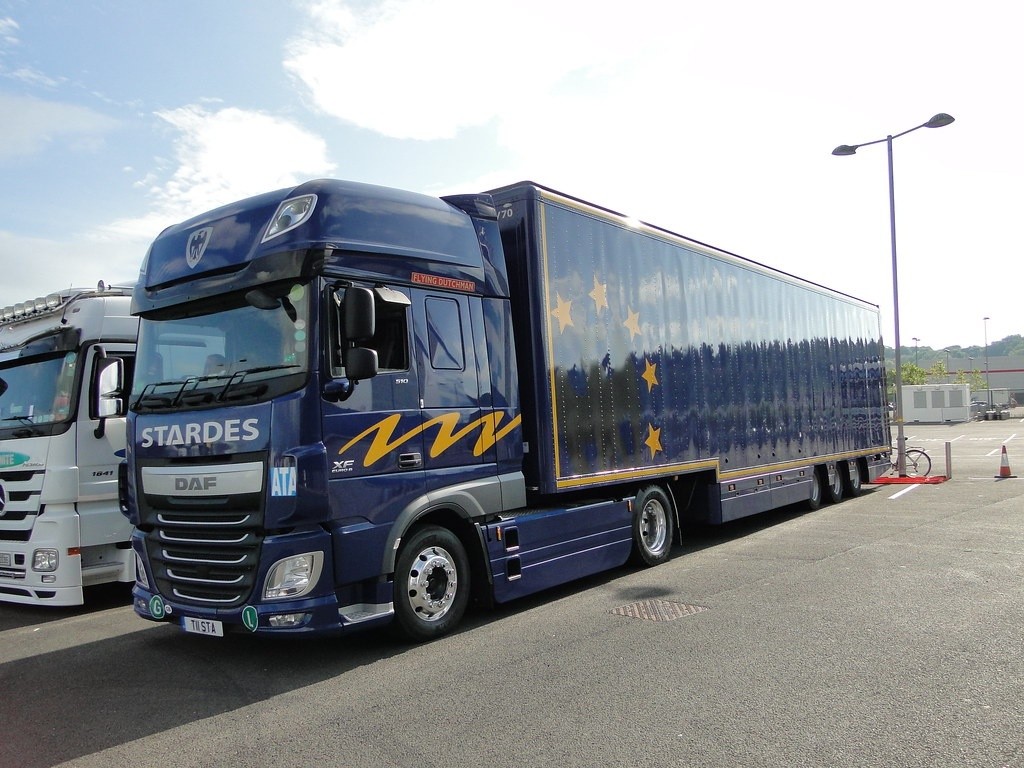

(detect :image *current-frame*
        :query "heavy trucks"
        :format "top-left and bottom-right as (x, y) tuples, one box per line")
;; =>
(0, 279), (140, 606)
(127, 179), (892, 642)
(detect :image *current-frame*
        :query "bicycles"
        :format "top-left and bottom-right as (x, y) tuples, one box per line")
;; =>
(890, 437), (931, 478)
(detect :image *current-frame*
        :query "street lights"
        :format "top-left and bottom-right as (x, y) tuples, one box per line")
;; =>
(984, 317), (990, 410)
(945, 349), (949, 374)
(969, 357), (973, 389)
(912, 338), (920, 367)
(831, 113), (955, 478)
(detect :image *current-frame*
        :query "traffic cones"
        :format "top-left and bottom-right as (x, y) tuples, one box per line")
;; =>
(995, 445), (1017, 477)
